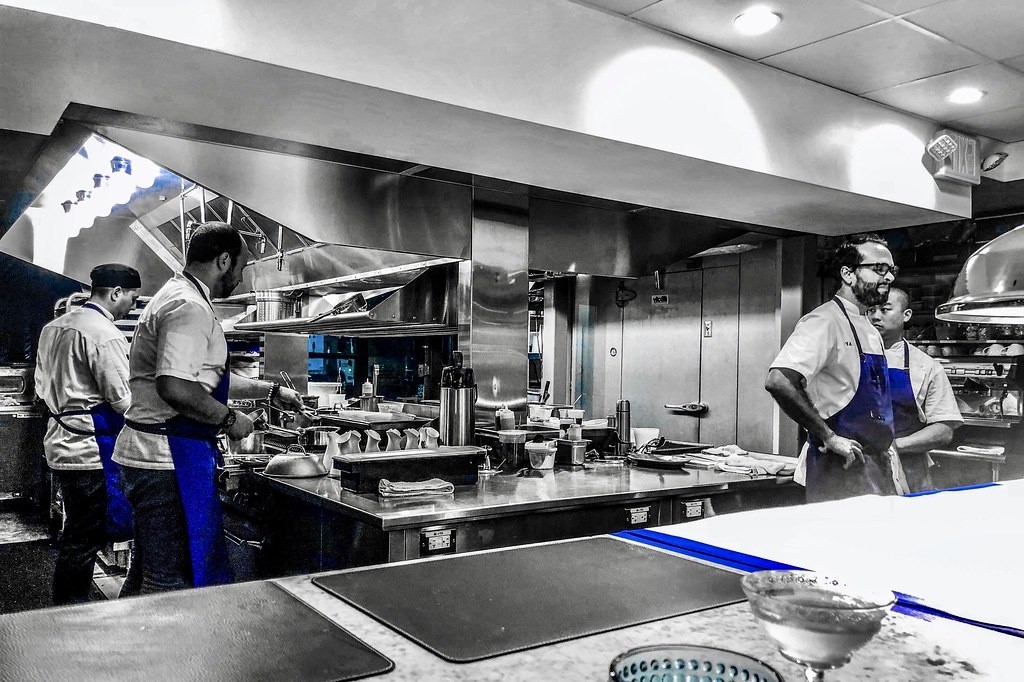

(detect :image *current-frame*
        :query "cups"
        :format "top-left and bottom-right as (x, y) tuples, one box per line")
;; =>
(634, 428), (659, 450)
(974, 342), (1024, 357)
(615, 400), (630, 456)
(498, 431), (527, 469)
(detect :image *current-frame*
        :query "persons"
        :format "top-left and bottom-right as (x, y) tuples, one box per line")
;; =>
(765, 230), (904, 506)
(867, 289), (965, 494)
(32, 262), (143, 604)
(107, 221), (303, 602)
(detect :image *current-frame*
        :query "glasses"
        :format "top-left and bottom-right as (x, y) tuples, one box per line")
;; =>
(847, 263), (899, 277)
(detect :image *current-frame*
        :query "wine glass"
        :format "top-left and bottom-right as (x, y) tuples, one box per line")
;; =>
(740, 568), (898, 682)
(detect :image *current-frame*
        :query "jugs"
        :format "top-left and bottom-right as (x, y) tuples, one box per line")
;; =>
(439, 351), (478, 447)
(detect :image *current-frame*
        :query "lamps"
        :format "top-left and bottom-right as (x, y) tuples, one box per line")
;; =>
(927, 135), (958, 162)
(981, 152), (1008, 172)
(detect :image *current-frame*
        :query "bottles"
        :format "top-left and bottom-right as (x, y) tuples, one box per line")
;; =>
(324, 428), (439, 475)
(495, 404), (505, 431)
(500, 405), (515, 430)
(362, 378), (372, 396)
(569, 424), (581, 441)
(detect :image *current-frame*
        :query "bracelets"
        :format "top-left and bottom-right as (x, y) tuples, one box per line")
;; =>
(266, 382), (281, 400)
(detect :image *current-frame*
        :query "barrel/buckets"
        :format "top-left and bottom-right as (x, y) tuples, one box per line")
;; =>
(529, 405), (555, 422)
(359, 396), (384, 412)
(559, 409), (585, 424)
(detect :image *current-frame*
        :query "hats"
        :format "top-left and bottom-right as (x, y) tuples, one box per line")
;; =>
(90, 263), (141, 288)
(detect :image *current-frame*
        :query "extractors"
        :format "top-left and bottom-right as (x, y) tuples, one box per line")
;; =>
(0, 127), (470, 332)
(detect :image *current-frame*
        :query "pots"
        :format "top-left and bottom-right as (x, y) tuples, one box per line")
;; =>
(268, 395), (319, 427)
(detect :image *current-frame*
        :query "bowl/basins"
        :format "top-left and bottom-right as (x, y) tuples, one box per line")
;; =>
(392, 403), (404, 412)
(377, 404), (391, 413)
(610, 645), (786, 682)
(247, 408), (268, 426)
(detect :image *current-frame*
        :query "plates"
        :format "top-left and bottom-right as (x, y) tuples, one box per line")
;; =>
(628, 453), (690, 469)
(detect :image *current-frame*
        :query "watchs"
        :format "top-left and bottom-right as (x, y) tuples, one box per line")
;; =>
(219, 407), (236, 427)
